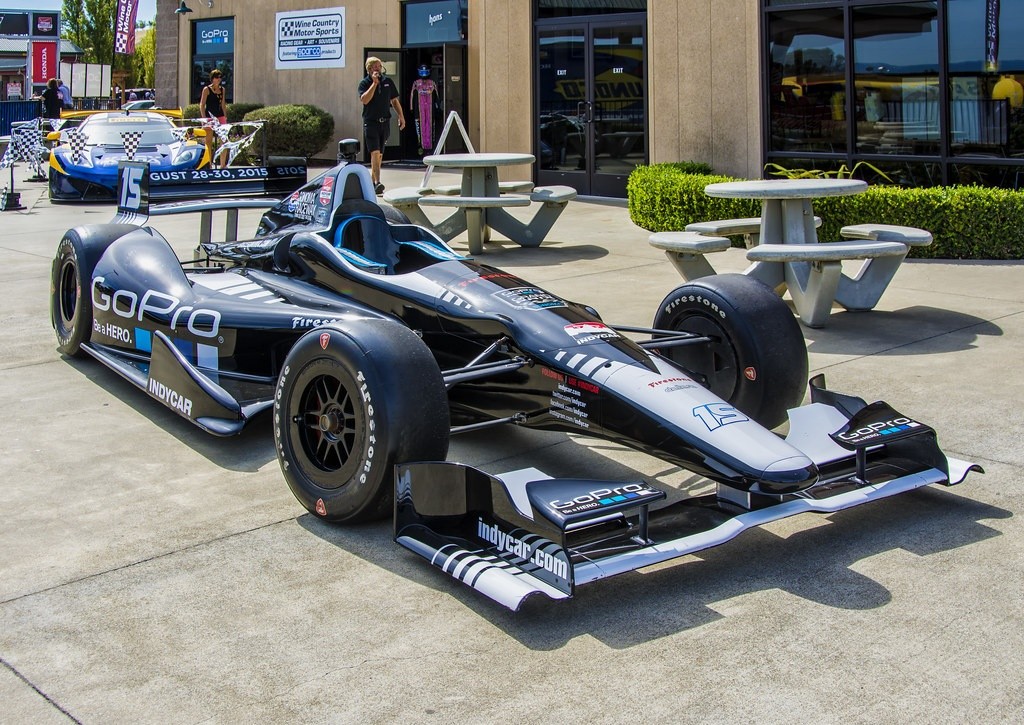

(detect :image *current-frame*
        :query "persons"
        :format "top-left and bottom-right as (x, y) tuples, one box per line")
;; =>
(410, 65), (440, 161)
(358, 57), (405, 194)
(130, 89), (137, 100)
(144, 89), (155, 100)
(200, 70), (228, 168)
(32, 78), (60, 120)
(57, 79), (72, 111)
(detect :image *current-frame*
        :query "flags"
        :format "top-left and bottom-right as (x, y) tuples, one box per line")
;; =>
(116, 0), (138, 52)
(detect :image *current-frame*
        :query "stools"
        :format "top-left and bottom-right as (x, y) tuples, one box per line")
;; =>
(417, 194), (531, 255)
(433, 180), (535, 244)
(649, 230), (786, 290)
(744, 239), (908, 331)
(832, 223), (934, 314)
(383, 185), (468, 242)
(685, 215), (822, 298)
(485, 185), (577, 248)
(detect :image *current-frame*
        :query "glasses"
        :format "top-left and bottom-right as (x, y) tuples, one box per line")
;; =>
(214, 74), (223, 78)
(371, 66), (387, 74)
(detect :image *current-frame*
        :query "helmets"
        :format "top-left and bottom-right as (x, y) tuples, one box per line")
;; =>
(418, 65), (431, 78)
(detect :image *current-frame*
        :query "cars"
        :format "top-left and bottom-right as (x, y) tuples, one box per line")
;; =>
(120, 100), (155, 112)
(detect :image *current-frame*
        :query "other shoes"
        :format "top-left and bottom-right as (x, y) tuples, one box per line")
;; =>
(373, 182), (385, 195)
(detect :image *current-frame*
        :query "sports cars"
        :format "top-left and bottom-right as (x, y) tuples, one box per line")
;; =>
(45, 108), (211, 203)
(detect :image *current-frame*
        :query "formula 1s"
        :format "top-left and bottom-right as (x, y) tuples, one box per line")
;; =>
(43, 138), (985, 616)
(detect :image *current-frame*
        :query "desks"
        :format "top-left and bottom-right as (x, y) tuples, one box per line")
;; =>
(704, 178), (869, 282)
(422, 153), (538, 225)
